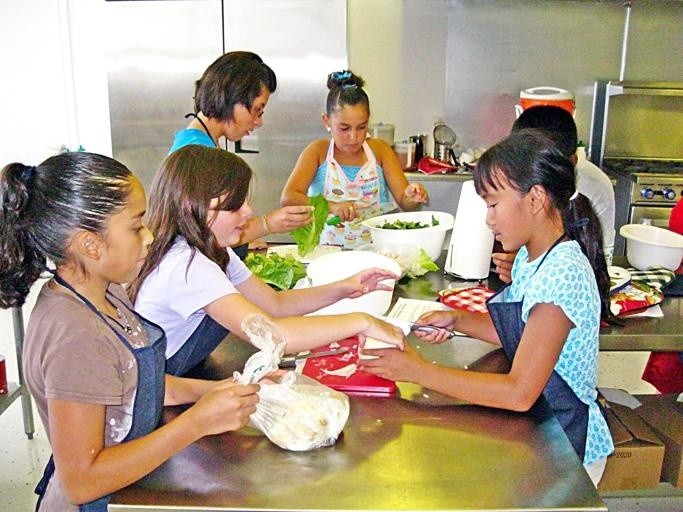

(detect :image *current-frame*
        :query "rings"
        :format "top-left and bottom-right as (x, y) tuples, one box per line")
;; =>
(349, 206), (355, 211)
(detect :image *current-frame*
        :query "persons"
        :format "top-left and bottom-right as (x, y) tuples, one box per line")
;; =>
(164, 49), (315, 257)
(640, 197), (683, 394)
(280, 70), (430, 248)
(490, 103), (615, 283)
(120, 145), (406, 377)
(361, 130), (614, 488)
(0, 153), (288, 510)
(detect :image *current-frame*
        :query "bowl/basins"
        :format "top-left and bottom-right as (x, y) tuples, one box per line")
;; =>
(617, 222), (683, 272)
(361, 211), (455, 274)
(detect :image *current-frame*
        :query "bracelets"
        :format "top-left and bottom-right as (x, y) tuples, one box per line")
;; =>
(260, 214), (271, 236)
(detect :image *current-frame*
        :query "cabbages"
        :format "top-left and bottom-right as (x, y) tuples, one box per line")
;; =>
(245, 254), (304, 291)
(289, 194), (328, 257)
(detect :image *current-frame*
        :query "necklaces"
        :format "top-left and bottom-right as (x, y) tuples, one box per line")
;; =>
(102, 292), (132, 334)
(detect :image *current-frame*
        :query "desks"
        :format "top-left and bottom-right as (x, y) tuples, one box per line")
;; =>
(591, 275), (683, 355)
(106, 231), (605, 512)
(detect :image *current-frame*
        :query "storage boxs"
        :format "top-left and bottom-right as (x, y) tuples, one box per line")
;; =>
(633, 394), (682, 491)
(588, 400), (665, 489)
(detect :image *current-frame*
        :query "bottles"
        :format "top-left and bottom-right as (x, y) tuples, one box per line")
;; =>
(367, 122), (395, 148)
(390, 139), (415, 174)
(412, 137), (423, 163)
